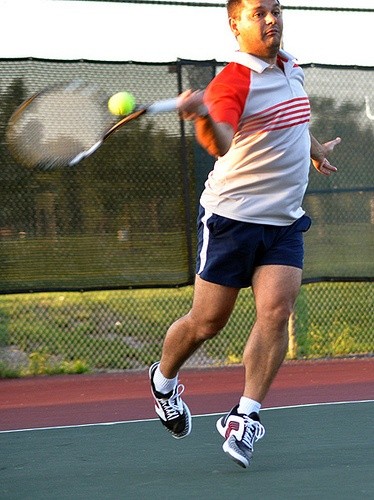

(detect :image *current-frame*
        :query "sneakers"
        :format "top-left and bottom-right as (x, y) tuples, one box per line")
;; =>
(215, 403), (265, 469)
(147, 361), (192, 440)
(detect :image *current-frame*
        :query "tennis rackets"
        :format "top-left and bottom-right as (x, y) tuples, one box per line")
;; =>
(4, 81), (177, 170)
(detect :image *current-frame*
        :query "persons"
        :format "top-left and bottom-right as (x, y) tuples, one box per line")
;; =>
(149, 0), (342, 469)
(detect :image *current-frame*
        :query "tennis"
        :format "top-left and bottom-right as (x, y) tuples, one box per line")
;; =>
(108, 92), (135, 116)
